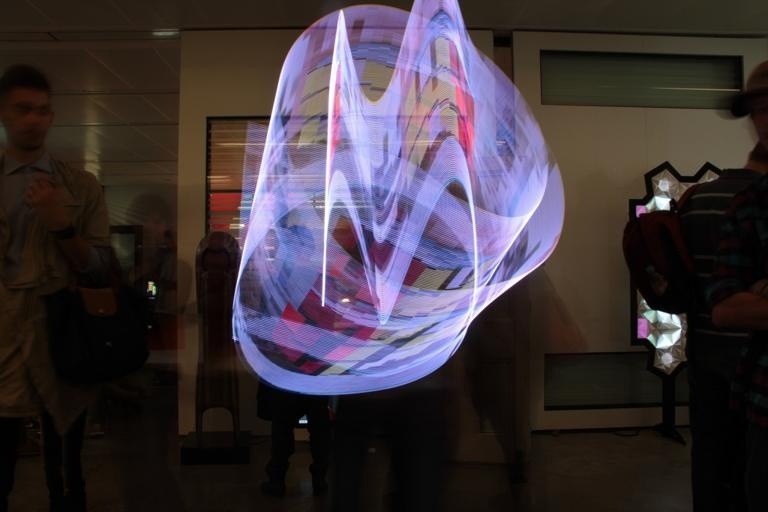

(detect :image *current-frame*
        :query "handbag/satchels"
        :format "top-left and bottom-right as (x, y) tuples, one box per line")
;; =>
(35, 242), (161, 388)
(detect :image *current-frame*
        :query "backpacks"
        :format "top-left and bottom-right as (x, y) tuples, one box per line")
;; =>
(619, 181), (711, 316)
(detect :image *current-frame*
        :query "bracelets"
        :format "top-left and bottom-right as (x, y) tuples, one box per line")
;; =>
(47, 223), (74, 240)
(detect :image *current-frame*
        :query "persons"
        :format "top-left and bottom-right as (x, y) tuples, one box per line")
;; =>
(1, 63), (122, 511)
(663, 59), (766, 512)
(330, 389), (453, 510)
(106, 191), (192, 511)
(251, 377), (331, 508)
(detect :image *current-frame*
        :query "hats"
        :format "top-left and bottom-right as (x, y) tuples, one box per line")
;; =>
(729, 57), (768, 118)
